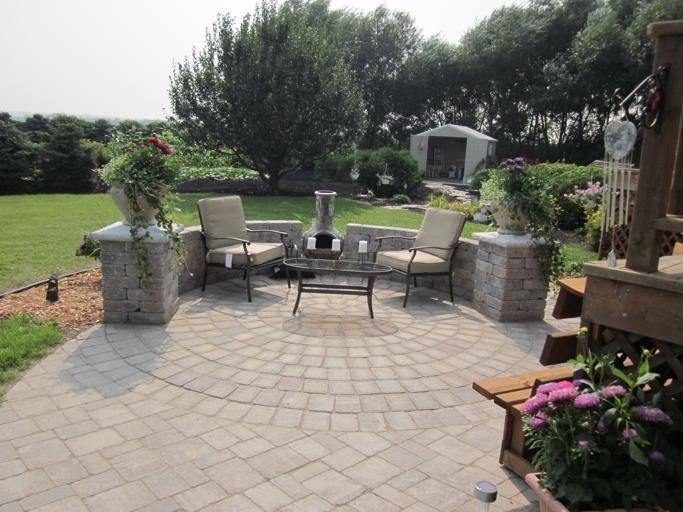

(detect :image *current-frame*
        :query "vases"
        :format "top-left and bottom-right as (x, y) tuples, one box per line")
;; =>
(480, 196), (532, 235)
(110, 184), (168, 226)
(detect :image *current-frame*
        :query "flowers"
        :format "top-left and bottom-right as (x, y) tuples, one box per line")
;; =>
(520, 323), (683, 512)
(479, 156), (569, 295)
(89, 135), (196, 290)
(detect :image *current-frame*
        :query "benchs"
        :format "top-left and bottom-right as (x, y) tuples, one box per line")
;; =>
(471, 367), (576, 480)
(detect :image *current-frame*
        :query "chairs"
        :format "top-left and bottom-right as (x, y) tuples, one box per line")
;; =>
(372, 207), (467, 308)
(197, 194), (292, 303)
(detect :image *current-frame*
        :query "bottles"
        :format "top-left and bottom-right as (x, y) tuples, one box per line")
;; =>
(607, 249), (617, 269)
